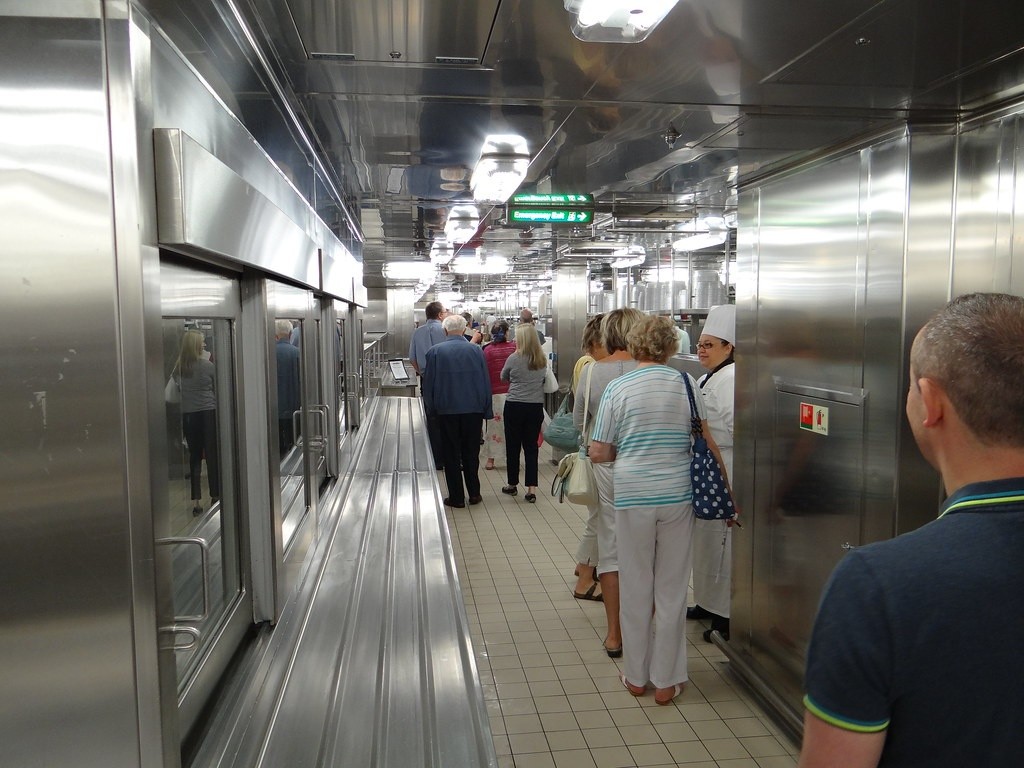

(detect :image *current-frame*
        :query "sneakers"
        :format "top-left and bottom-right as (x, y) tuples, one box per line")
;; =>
(525, 493), (536, 503)
(502, 485), (517, 496)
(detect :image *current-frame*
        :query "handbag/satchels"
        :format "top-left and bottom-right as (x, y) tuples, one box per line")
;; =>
(164, 361), (184, 415)
(538, 407), (552, 448)
(541, 391), (584, 452)
(679, 372), (736, 519)
(550, 444), (599, 505)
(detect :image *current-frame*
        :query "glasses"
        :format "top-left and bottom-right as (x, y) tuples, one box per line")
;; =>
(696, 343), (713, 349)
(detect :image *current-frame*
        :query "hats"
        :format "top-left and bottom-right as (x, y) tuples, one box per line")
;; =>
(702, 304), (736, 348)
(486, 316), (496, 325)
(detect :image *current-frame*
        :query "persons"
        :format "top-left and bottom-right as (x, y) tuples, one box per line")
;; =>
(409, 300), (547, 507)
(172, 319), (300, 516)
(686, 304), (736, 642)
(588, 315), (737, 704)
(570, 307), (666, 657)
(799, 293), (1024, 768)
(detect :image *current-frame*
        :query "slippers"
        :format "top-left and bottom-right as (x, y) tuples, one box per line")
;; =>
(620, 675), (639, 696)
(655, 684), (684, 705)
(602, 634), (622, 658)
(574, 582), (604, 601)
(575, 567), (601, 582)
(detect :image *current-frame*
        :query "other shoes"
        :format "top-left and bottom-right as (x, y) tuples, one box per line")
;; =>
(687, 606), (712, 620)
(444, 498), (465, 508)
(193, 507), (203, 516)
(485, 458), (494, 470)
(210, 498), (219, 507)
(469, 495), (482, 504)
(703, 628), (730, 642)
